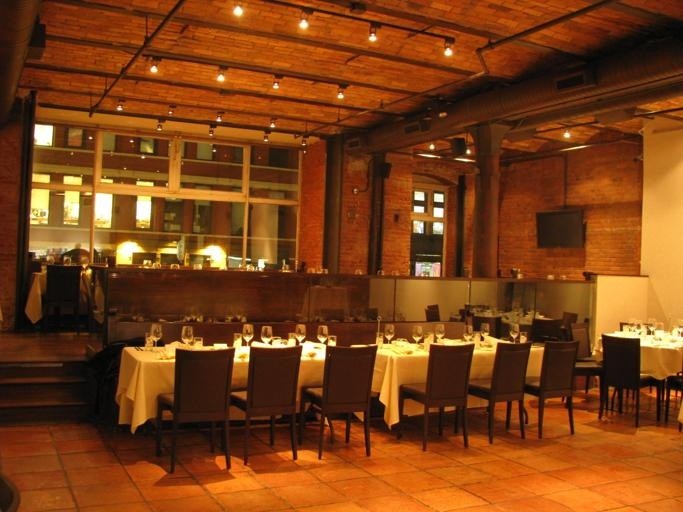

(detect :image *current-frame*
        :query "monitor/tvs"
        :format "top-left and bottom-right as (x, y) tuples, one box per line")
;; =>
(536, 208), (586, 248)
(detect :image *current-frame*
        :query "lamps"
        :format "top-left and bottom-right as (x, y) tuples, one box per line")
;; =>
(369, 26), (377, 42)
(299, 11), (308, 30)
(337, 86), (344, 99)
(218, 67), (226, 82)
(150, 58), (160, 73)
(272, 76), (280, 89)
(90, 100), (464, 152)
(443, 41), (453, 57)
(233, 1), (243, 18)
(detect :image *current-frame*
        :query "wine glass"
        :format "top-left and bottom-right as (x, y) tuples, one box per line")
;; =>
(383, 324), (394, 348)
(510, 322), (520, 346)
(624, 316), (681, 344)
(150, 323), (160, 352)
(434, 323), (445, 342)
(410, 325), (423, 347)
(240, 324), (255, 350)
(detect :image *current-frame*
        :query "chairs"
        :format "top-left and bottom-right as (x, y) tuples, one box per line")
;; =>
(505, 336), (585, 446)
(228, 340), (312, 465)
(460, 336), (534, 447)
(17, 244), (291, 332)
(151, 345), (241, 479)
(296, 337), (383, 466)
(419, 295), (584, 339)
(392, 336), (482, 453)
(565, 317), (681, 435)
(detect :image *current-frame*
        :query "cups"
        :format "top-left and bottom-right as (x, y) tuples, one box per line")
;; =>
(519, 331), (527, 345)
(317, 324), (328, 346)
(463, 325), (472, 342)
(479, 321), (490, 339)
(354, 269), (361, 275)
(192, 337), (206, 349)
(374, 332), (384, 350)
(259, 325), (272, 345)
(232, 333), (242, 349)
(421, 332), (435, 352)
(288, 332), (296, 349)
(180, 326), (194, 345)
(43, 247), (329, 275)
(470, 331), (482, 348)
(376, 270), (383, 275)
(272, 336), (281, 346)
(143, 332), (154, 352)
(294, 322), (307, 348)
(326, 335), (336, 348)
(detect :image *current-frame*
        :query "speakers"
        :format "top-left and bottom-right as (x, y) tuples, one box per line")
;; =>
(369, 161), (392, 178)
